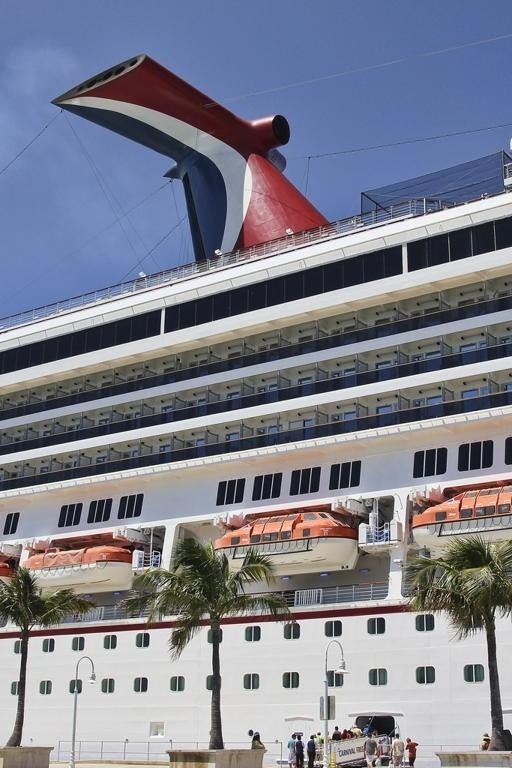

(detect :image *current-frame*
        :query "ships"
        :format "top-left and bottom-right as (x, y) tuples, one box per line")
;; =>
(1, 54), (511, 767)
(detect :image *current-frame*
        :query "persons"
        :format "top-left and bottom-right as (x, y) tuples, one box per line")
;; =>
(307, 723), (419, 768)
(251, 732), (265, 749)
(295, 735), (305, 767)
(287, 734), (297, 768)
(479, 732), (491, 751)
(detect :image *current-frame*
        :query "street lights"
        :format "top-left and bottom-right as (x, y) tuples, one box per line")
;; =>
(322, 640), (349, 768)
(69, 656), (96, 768)
